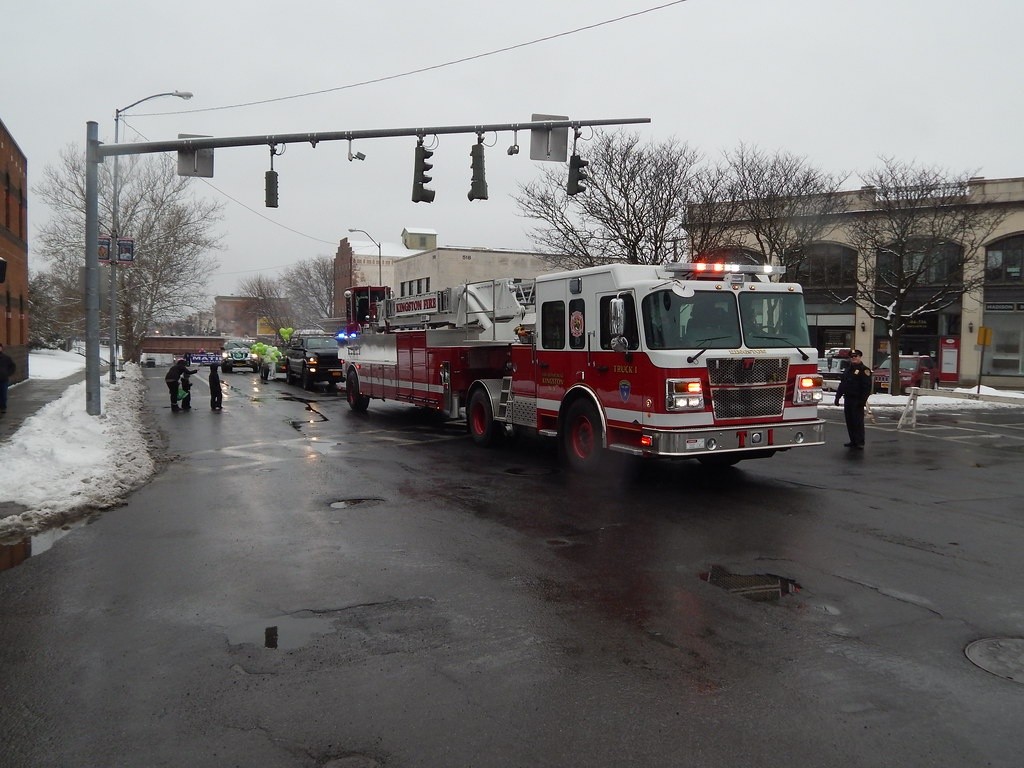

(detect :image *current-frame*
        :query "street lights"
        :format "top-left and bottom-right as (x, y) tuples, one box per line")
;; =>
(348, 228), (382, 288)
(111, 91), (192, 387)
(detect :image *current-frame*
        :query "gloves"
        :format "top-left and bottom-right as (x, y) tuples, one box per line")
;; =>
(834, 397), (840, 406)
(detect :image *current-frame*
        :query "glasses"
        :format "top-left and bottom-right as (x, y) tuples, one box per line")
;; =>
(850, 354), (859, 357)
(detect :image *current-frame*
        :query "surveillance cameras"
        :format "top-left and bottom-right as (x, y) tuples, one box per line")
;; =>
(355, 152), (366, 161)
(507, 146), (515, 155)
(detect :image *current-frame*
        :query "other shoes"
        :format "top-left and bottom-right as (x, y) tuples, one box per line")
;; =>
(176, 388), (188, 401)
(843, 440), (856, 447)
(858, 442), (865, 450)
(172, 407), (181, 411)
(215, 406), (223, 411)
(182, 406), (192, 409)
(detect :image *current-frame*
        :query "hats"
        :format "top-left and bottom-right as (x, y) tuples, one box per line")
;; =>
(848, 350), (862, 356)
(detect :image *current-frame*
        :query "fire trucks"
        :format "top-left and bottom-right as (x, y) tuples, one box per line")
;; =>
(333, 263), (827, 474)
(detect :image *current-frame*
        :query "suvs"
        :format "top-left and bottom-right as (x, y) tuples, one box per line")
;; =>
(870, 355), (940, 395)
(220, 339), (260, 374)
(817, 347), (853, 379)
(284, 334), (346, 390)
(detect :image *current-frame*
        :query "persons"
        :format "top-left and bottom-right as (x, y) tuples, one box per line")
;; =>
(165, 359), (200, 412)
(260, 360), (276, 384)
(183, 352), (191, 366)
(209, 363), (223, 410)
(180, 373), (193, 409)
(0, 343), (16, 412)
(834, 349), (873, 450)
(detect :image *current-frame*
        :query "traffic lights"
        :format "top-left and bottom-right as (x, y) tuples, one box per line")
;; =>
(412, 146), (436, 203)
(566, 155), (589, 194)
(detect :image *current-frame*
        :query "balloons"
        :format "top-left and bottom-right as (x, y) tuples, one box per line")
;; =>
(279, 328), (293, 340)
(250, 342), (282, 363)
